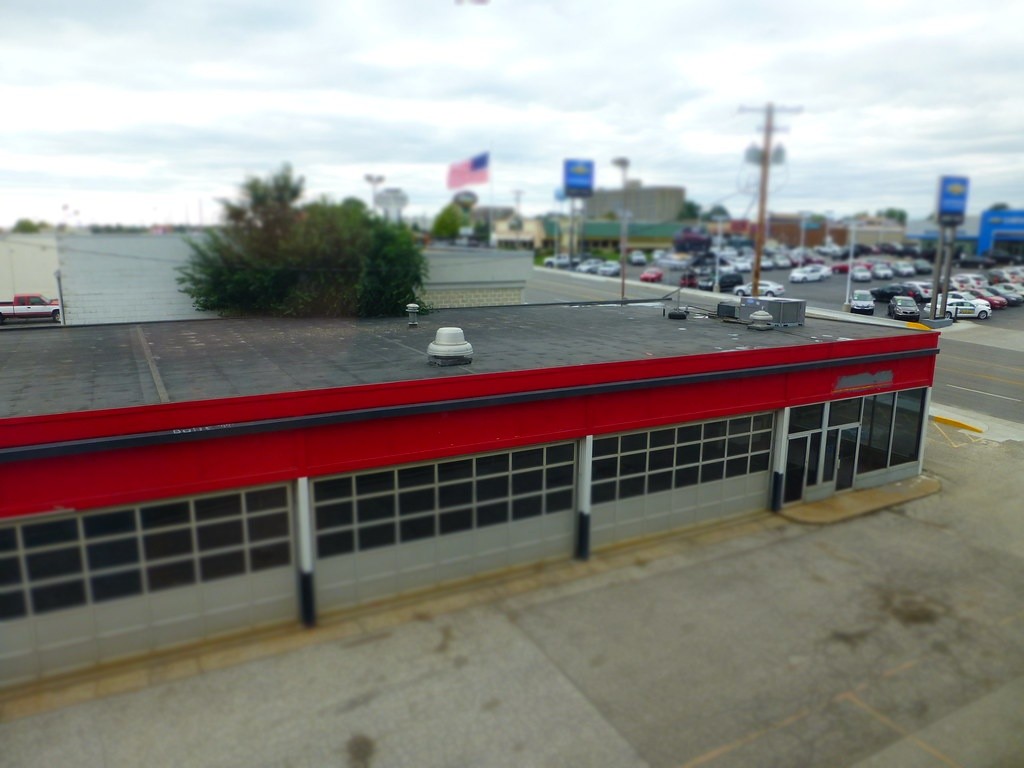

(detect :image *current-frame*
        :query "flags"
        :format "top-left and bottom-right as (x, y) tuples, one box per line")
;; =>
(446, 153), (489, 188)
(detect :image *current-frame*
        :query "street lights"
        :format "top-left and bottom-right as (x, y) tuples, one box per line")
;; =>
(610, 156), (632, 302)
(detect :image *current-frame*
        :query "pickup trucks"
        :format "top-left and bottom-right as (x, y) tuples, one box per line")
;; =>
(0, 294), (61, 323)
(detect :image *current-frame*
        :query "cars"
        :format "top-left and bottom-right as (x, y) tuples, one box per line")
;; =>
(888, 296), (922, 322)
(866, 264), (1024, 319)
(598, 260), (622, 278)
(699, 272), (743, 292)
(640, 267), (663, 282)
(576, 258), (602, 274)
(544, 252), (581, 270)
(848, 290), (876, 316)
(733, 280), (784, 297)
(632, 232), (934, 282)
